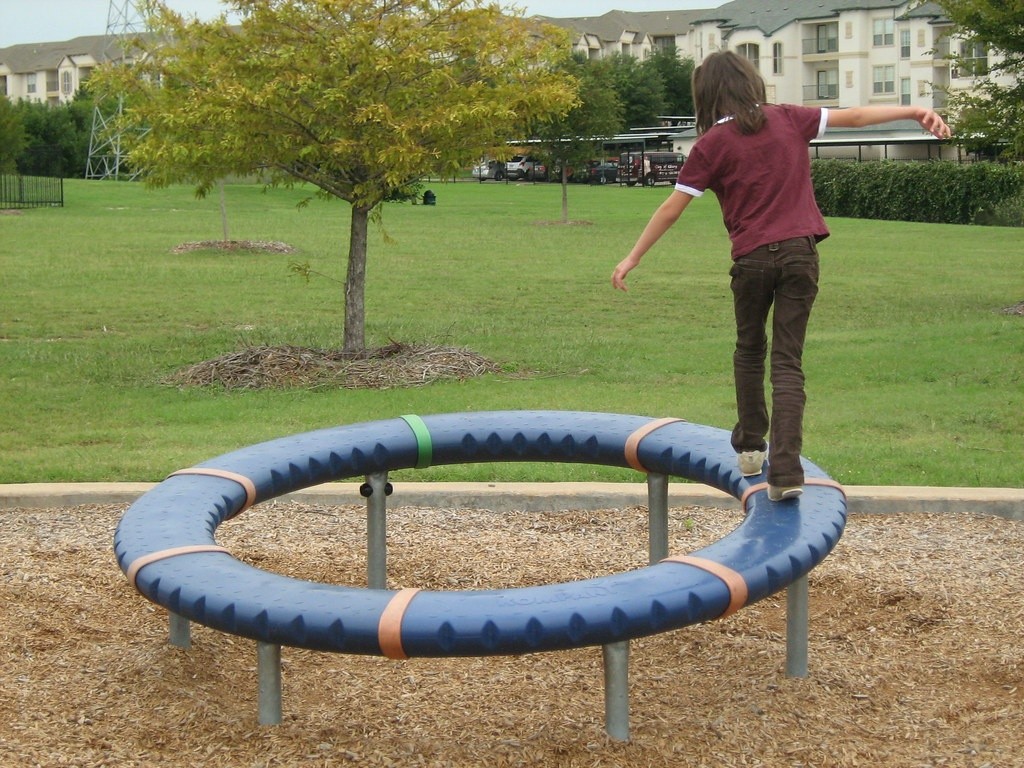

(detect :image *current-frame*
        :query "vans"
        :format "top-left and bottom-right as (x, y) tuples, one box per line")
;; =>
(614, 152), (689, 186)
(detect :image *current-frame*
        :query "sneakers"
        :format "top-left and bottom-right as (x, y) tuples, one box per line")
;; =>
(738, 450), (767, 476)
(768, 483), (803, 502)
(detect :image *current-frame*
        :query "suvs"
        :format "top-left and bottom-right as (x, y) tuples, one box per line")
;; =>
(549, 155), (581, 184)
(502, 154), (536, 181)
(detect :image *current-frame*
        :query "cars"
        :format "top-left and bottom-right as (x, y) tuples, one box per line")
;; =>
(575, 161), (618, 185)
(471, 159), (507, 182)
(527, 158), (549, 181)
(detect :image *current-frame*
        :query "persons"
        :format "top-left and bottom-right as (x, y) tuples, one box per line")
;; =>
(611, 51), (951, 501)
(641, 157), (651, 186)
